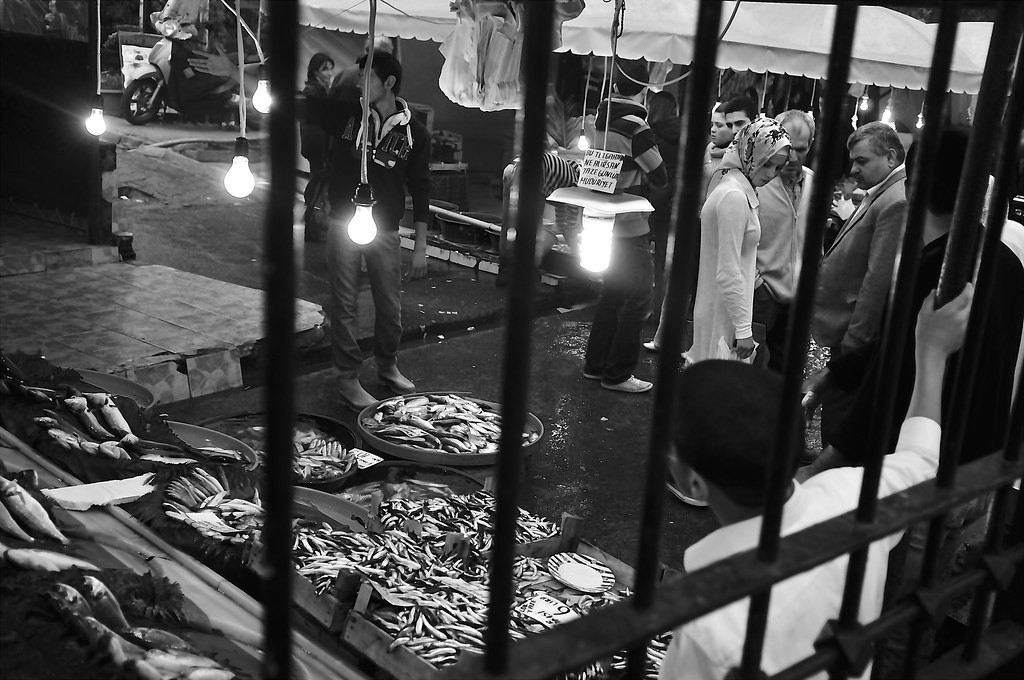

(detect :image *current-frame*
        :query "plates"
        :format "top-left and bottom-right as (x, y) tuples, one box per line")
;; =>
(547, 552), (615, 593)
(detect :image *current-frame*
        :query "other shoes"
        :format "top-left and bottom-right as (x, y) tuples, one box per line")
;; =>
(798, 445), (820, 464)
(663, 475), (708, 507)
(600, 374), (653, 392)
(642, 340), (655, 350)
(581, 366), (603, 379)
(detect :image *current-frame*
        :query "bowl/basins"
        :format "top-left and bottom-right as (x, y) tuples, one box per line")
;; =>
(399, 197), (579, 275)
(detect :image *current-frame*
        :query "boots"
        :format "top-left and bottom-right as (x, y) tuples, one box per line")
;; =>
(337, 376), (379, 413)
(376, 357), (416, 395)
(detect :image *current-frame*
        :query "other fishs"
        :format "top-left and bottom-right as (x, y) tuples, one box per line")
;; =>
(0, 386), (676, 680)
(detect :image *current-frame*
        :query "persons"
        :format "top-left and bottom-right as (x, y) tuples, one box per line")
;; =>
(754, 110), (819, 464)
(647, 91), (681, 323)
(187, 39), (431, 408)
(665, 116), (793, 504)
(596, 80), (613, 107)
(300, 53), (335, 275)
(546, 68), (584, 150)
(582, 61), (667, 392)
(810, 121), (906, 451)
(326, 35), (393, 285)
(656, 283), (974, 680)
(700, 97), (760, 211)
(796, 123), (1023, 680)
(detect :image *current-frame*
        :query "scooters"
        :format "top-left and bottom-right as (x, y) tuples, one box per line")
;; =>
(118, 0), (269, 130)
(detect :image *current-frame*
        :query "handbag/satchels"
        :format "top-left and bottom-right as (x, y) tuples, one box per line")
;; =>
(303, 174), (331, 245)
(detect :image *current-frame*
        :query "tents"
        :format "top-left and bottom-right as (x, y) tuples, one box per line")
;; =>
(300, 1), (984, 93)
